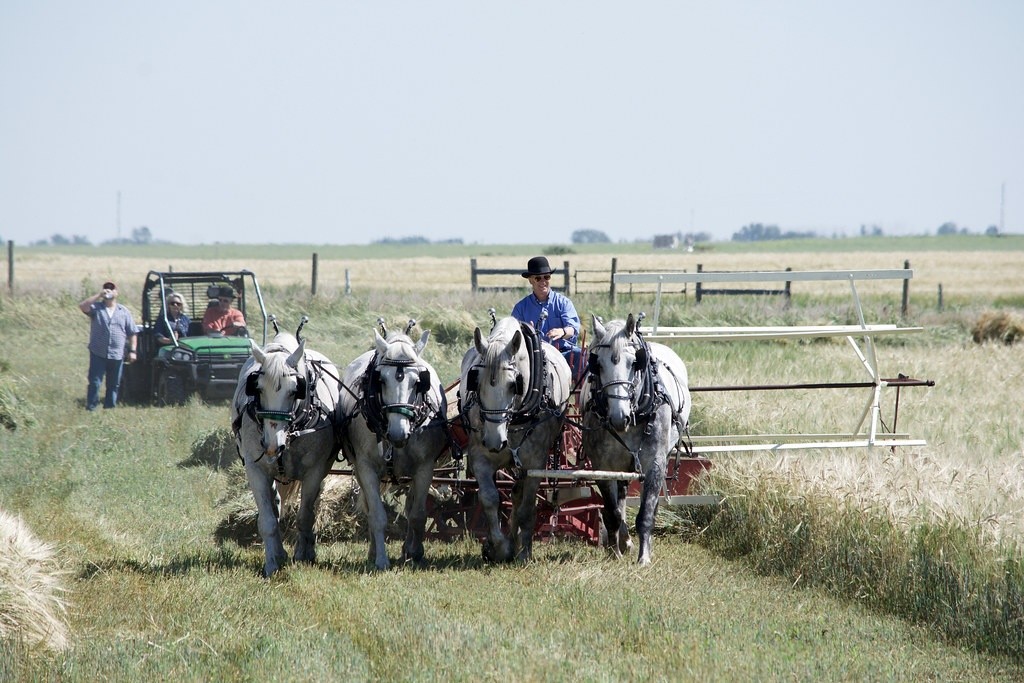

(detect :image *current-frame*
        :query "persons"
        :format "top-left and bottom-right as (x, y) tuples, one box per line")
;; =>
(201, 286), (246, 337)
(512, 256), (589, 378)
(78, 281), (140, 412)
(153, 286), (191, 346)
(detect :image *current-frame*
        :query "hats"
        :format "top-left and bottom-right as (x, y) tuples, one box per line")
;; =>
(103, 280), (116, 286)
(522, 256), (556, 278)
(217, 286), (236, 298)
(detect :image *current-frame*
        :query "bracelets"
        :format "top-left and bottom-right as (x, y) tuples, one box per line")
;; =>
(128, 349), (137, 354)
(561, 327), (567, 339)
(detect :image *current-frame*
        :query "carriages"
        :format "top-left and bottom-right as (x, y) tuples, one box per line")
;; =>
(230, 312), (715, 580)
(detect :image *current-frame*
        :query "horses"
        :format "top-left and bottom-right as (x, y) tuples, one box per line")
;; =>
(462, 312), (692, 566)
(232, 330), (449, 583)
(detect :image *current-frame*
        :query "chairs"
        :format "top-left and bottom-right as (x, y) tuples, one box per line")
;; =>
(207, 286), (220, 308)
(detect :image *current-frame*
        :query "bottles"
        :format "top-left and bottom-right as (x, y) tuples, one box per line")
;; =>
(105, 293), (112, 298)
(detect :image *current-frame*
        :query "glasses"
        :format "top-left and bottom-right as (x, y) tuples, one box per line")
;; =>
(169, 302), (182, 306)
(530, 274), (551, 282)
(217, 297), (228, 303)
